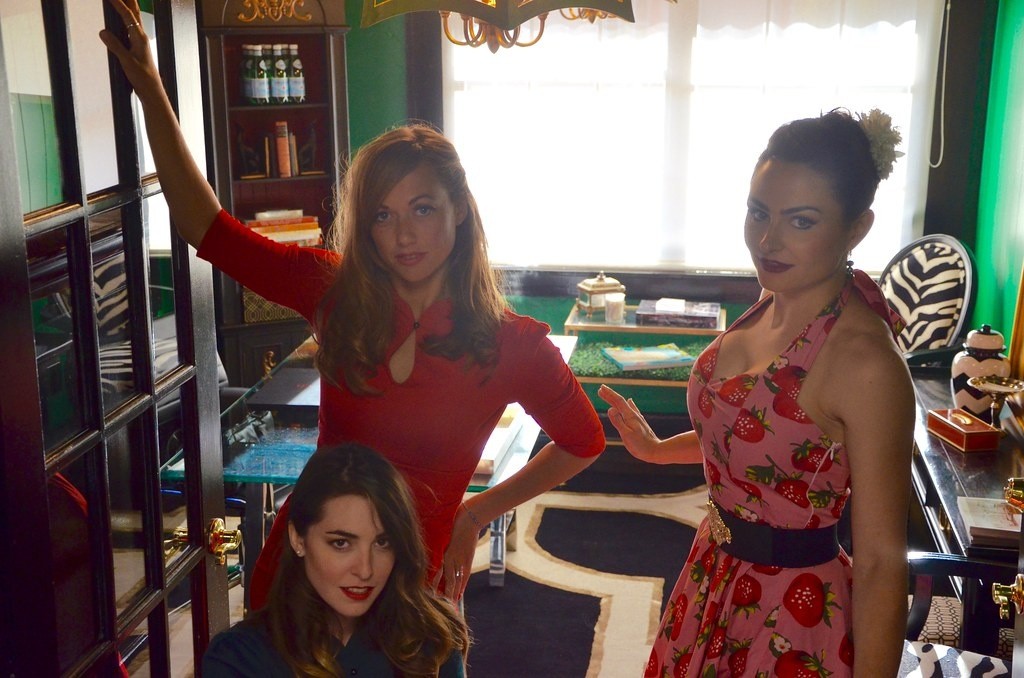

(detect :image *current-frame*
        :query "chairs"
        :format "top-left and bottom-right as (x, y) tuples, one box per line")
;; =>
(862, 230), (975, 362)
(44, 229), (228, 403)
(888, 553), (1024, 659)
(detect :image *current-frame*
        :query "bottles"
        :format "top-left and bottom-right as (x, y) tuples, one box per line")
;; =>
(241, 43), (306, 105)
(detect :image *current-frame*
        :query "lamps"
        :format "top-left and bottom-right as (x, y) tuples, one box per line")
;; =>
(358, 0), (638, 57)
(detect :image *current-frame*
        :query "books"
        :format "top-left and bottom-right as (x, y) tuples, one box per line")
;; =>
(963, 521), (1023, 552)
(956, 495), (1023, 541)
(634, 298), (721, 329)
(599, 342), (697, 371)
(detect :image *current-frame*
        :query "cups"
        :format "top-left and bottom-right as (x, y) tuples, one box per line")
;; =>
(604, 292), (625, 324)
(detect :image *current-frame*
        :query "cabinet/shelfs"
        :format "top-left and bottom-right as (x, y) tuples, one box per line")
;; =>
(198, 0), (357, 393)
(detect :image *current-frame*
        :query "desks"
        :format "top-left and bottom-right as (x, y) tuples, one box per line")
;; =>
(891, 363), (1024, 604)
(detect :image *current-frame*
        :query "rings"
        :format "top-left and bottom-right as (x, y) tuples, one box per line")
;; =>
(126, 22), (140, 29)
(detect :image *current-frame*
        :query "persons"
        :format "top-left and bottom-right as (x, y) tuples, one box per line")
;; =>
(597, 108), (917, 678)
(193, 445), (472, 678)
(98, 0), (608, 643)
(47, 470), (130, 677)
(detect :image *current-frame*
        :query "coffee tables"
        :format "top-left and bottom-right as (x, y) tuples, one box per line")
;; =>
(157, 324), (576, 591)
(565, 299), (729, 349)
